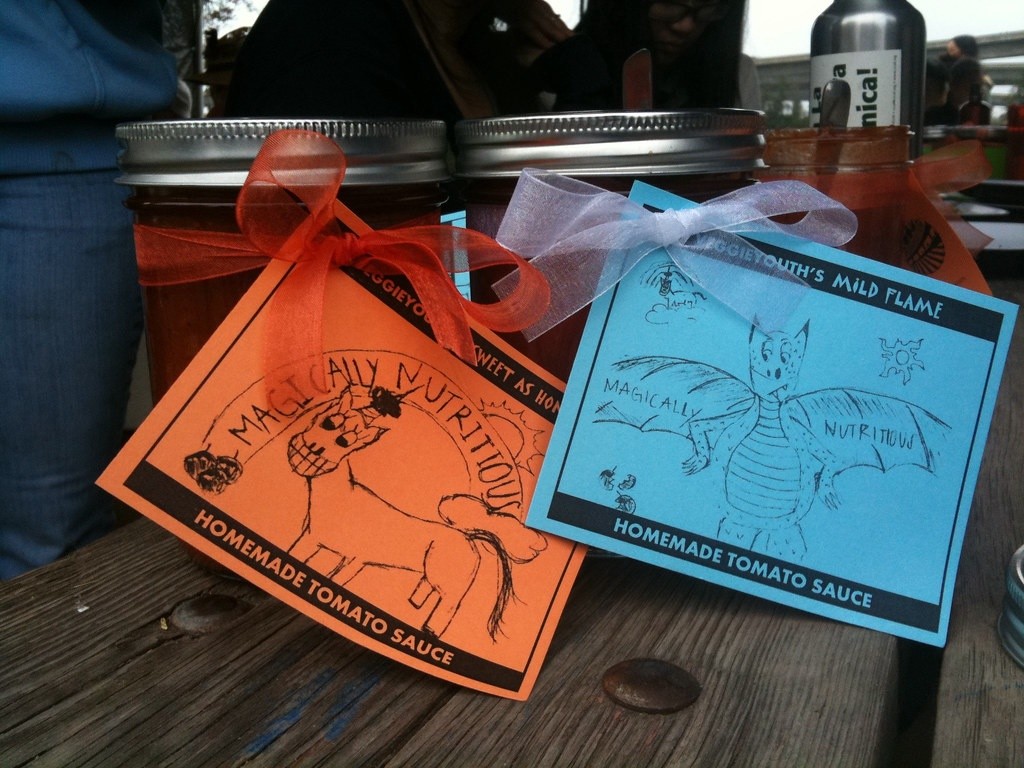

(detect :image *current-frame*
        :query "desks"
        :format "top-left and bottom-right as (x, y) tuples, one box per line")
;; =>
(1, 281), (1024, 768)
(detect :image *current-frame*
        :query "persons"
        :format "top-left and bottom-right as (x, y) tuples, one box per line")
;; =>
(926, 37), (990, 126)
(223, 0), (743, 142)
(0, 0), (175, 583)
